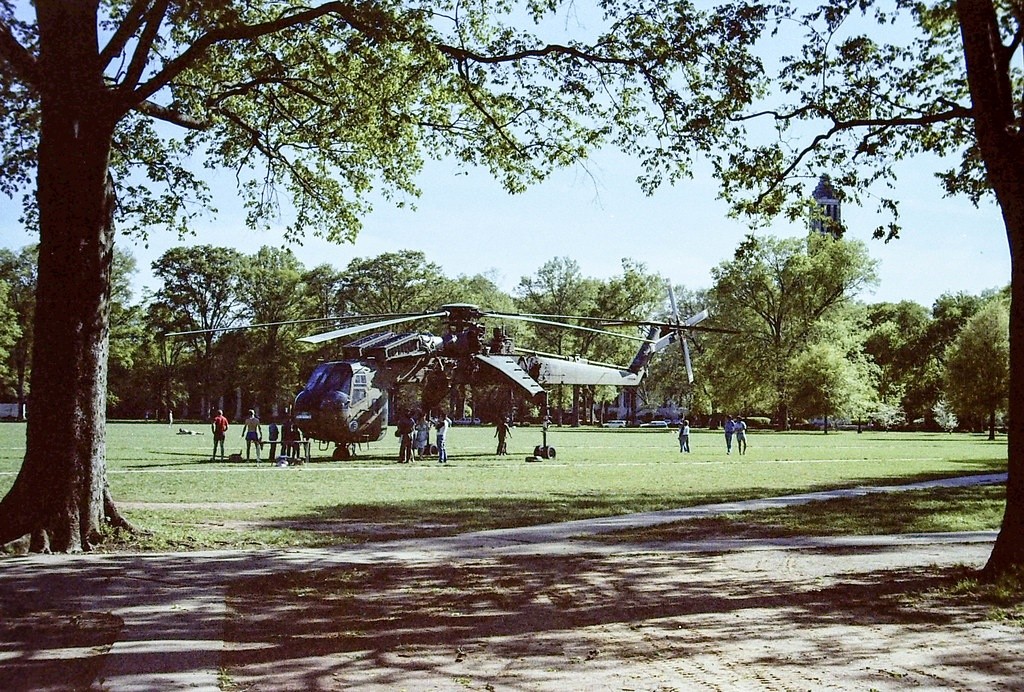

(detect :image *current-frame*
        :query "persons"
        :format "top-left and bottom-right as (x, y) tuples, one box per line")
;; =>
(269, 417), (279, 461)
(435, 414), (449, 463)
(725, 415), (736, 455)
(290, 425), (302, 460)
(281, 414), (292, 457)
(678, 419), (690, 453)
(143, 408), (173, 428)
(242, 409), (263, 463)
(415, 414), (430, 459)
(210, 409), (229, 462)
(494, 419), (512, 454)
(734, 416), (747, 455)
(397, 409), (416, 463)
(175, 428), (204, 435)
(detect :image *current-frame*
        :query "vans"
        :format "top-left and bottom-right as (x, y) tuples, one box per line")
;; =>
(454, 417), (481, 425)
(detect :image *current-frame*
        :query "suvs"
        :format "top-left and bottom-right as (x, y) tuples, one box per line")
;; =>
(639, 421), (670, 428)
(602, 420), (628, 428)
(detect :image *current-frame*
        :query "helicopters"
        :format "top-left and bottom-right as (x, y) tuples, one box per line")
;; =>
(159, 286), (741, 459)
